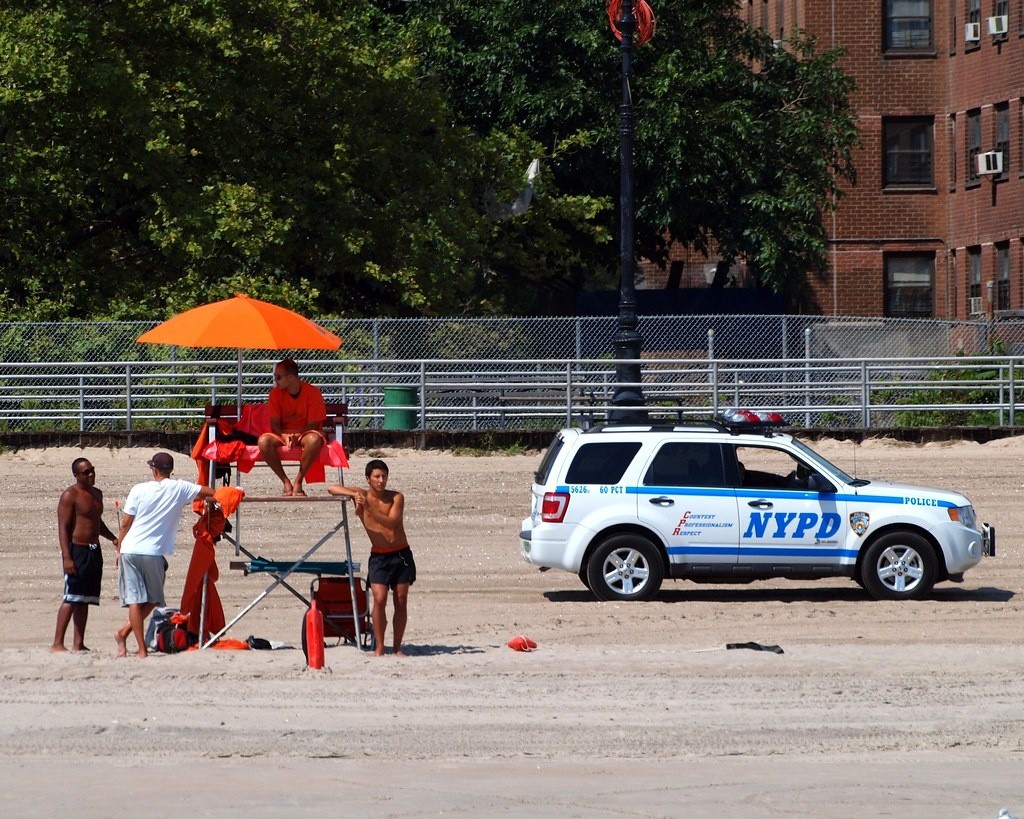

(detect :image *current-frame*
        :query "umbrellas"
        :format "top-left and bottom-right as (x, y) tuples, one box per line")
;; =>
(136, 293), (342, 556)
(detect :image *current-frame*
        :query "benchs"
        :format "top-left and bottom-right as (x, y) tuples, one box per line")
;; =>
(206, 403), (349, 496)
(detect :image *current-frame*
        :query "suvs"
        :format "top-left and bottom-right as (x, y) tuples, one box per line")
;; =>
(517, 409), (996, 601)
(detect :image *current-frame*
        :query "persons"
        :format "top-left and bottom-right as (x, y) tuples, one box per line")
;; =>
(113, 453), (215, 658)
(257, 360), (327, 496)
(50, 458), (118, 652)
(327, 460), (417, 655)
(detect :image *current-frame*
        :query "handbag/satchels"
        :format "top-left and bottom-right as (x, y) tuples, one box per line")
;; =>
(246, 636), (272, 651)
(157, 612), (190, 653)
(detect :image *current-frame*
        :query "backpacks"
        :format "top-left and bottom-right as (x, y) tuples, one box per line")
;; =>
(146, 607), (182, 652)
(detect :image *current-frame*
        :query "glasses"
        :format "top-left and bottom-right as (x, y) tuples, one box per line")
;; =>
(76, 467), (95, 475)
(150, 465), (155, 469)
(274, 372), (294, 381)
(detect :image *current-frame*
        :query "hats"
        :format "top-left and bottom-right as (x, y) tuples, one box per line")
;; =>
(147, 453), (173, 468)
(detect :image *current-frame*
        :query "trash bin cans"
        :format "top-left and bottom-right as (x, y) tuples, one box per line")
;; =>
(384, 387), (416, 429)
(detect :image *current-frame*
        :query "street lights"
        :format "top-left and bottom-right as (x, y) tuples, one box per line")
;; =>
(605, 0), (651, 426)
